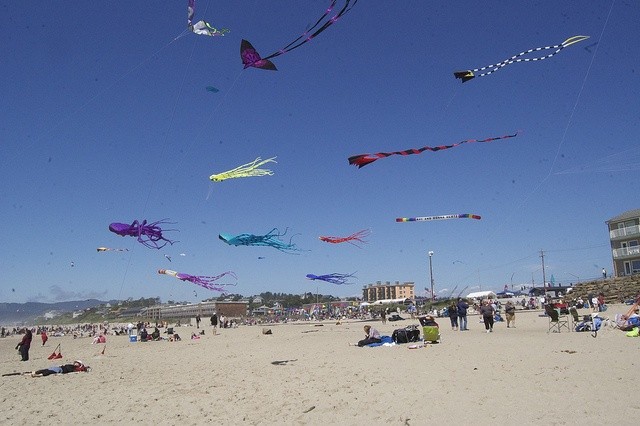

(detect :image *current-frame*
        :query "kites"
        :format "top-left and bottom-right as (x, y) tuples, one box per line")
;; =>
(395, 213), (482, 224)
(108, 218), (180, 249)
(319, 228), (369, 249)
(96, 247), (128, 254)
(241, 0), (358, 71)
(155, 267), (239, 293)
(454, 34), (594, 84)
(348, 131), (520, 168)
(304, 270), (356, 285)
(209, 156), (281, 183)
(219, 226), (312, 257)
(188, 0), (232, 38)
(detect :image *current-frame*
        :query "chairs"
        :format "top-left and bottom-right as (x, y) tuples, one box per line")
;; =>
(573, 315), (591, 331)
(550, 315), (569, 333)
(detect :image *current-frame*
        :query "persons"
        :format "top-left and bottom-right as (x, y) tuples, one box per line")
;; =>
(31, 360), (84, 377)
(419, 315), (438, 325)
(602, 266), (608, 279)
(457, 298), (469, 330)
(520, 292), (640, 318)
(354, 297), (449, 318)
(354, 326), (380, 346)
(480, 297), (494, 333)
(448, 302), (459, 331)
(504, 299), (517, 328)
